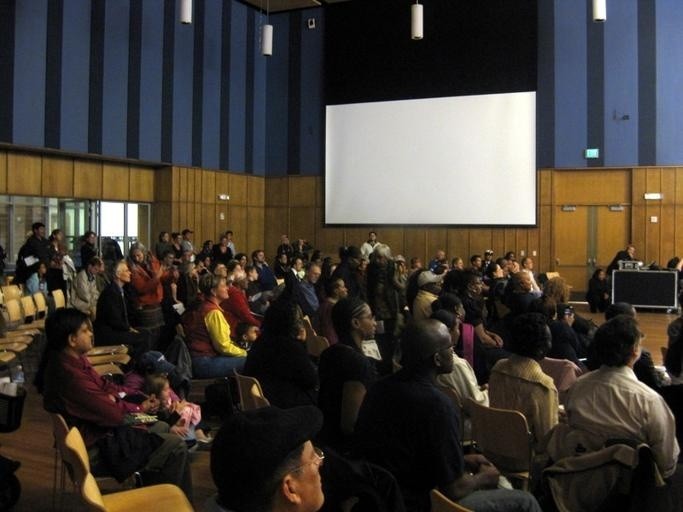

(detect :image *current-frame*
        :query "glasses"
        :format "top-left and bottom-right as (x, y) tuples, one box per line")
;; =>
(435, 344), (455, 352)
(288, 446), (325, 475)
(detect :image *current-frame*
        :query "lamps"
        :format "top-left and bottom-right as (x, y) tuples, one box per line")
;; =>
(260, 0), (273, 58)
(179, 0), (194, 24)
(409, 0), (428, 43)
(591, 0), (607, 25)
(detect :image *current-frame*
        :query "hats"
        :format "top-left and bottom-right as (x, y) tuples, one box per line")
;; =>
(138, 350), (176, 373)
(348, 246), (365, 261)
(209, 406), (323, 489)
(416, 270), (441, 287)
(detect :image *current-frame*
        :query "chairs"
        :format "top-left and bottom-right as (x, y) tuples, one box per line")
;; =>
(0, 272), (683, 510)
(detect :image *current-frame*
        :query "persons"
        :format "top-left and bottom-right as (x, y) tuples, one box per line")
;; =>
(0, 220), (683, 512)
